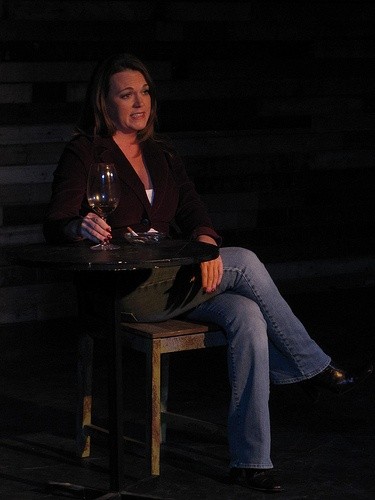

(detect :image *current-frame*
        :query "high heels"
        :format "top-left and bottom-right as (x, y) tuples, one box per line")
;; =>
(233, 462), (288, 491)
(315, 362), (368, 398)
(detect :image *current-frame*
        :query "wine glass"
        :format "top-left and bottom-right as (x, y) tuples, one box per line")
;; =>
(87, 164), (120, 250)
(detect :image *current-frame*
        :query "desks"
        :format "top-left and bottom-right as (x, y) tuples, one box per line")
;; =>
(8, 235), (222, 500)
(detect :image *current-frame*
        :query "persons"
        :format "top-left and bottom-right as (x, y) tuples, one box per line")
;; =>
(40, 54), (375, 492)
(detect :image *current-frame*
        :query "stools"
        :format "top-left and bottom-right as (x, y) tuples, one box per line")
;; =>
(74, 267), (230, 476)
